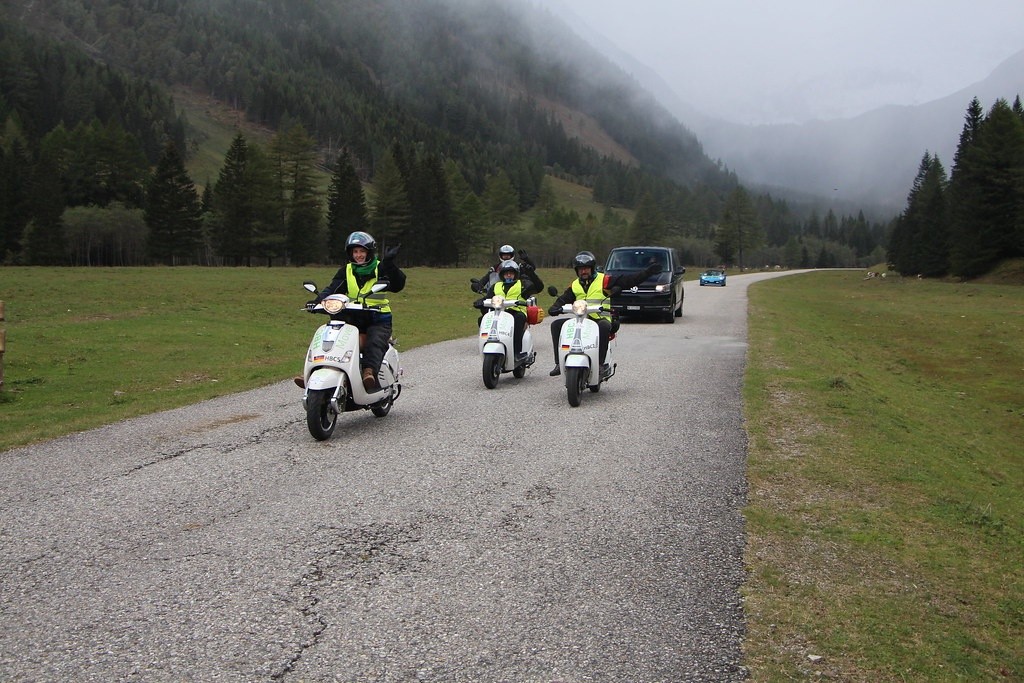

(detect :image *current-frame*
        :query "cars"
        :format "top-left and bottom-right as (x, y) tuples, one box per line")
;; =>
(699, 269), (727, 287)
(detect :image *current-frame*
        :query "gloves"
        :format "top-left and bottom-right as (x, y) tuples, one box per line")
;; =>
(384, 242), (401, 263)
(305, 301), (317, 315)
(518, 249), (529, 263)
(520, 262), (534, 274)
(471, 282), (482, 292)
(473, 299), (484, 309)
(643, 263), (663, 278)
(548, 305), (560, 317)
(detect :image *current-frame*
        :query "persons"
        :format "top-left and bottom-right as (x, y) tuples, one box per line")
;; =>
(645, 256), (664, 270)
(294, 232), (406, 392)
(471, 245), (545, 360)
(548, 251), (623, 375)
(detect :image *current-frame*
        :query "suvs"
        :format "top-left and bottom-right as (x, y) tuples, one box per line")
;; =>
(596, 246), (686, 324)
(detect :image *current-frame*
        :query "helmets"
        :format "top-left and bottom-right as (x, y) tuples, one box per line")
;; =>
(497, 245), (515, 262)
(344, 231), (375, 266)
(497, 260), (519, 284)
(573, 250), (597, 277)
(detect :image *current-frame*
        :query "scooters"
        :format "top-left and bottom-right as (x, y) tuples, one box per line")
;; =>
(300, 279), (402, 440)
(470, 278), (537, 389)
(548, 285), (625, 406)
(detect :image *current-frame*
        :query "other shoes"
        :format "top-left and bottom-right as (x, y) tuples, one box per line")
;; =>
(362, 367), (375, 391)
(294, 375), (305, 390)
(550, 364), (561, 376)
